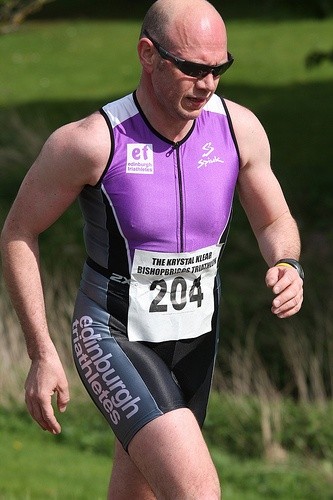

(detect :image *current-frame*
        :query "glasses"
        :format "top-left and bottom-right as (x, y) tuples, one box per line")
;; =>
(143, 29), (234, 76)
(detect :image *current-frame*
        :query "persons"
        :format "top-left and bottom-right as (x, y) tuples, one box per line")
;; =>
(0, 0), (304, 500)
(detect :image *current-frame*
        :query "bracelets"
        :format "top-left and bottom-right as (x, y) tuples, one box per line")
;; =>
(273, 258), (303, 278)
